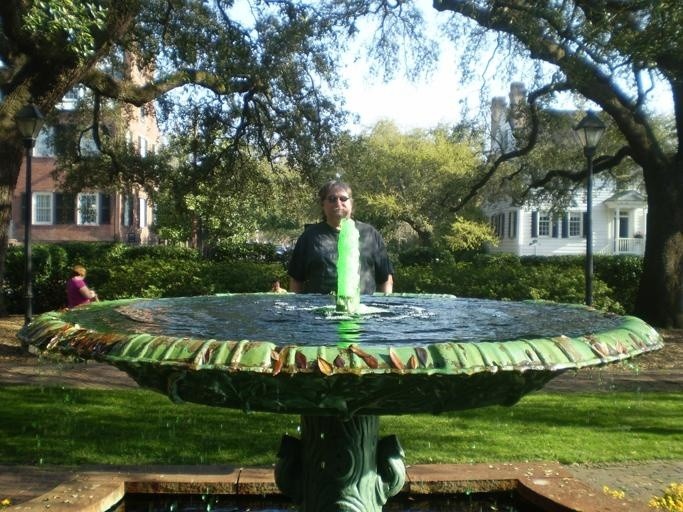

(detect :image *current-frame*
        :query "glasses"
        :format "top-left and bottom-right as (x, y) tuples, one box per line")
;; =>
(327, 195), (351, 202)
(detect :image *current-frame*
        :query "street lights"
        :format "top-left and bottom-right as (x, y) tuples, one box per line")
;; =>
(14, 102), (45, 353)
(573, 109), (606, 307)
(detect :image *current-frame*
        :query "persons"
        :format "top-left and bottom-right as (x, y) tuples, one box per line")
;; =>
(269, 279), (287, 293)
(65, 264), (94, 306)
(286, 179), (394, 295)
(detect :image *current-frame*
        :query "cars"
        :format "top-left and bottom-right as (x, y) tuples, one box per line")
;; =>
(265, 244), (287, 259)
